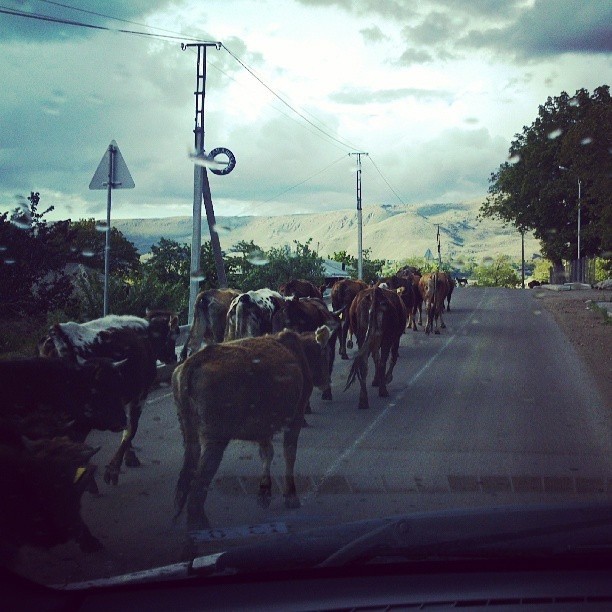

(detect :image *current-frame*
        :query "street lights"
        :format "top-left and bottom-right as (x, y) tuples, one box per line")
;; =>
(556, 164), (581, 283)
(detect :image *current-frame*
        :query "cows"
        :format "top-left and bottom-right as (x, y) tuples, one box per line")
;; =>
(35, 307), (180, 487)
(0, 437), (105, 553)
(331, 279), (375, 360)
(444, 272), (454, 311)
(267, 294), (349, 400)
(171, 326), (332, 532)
(372, 275), (417, 332)
(455, 276), (467, 286)
(419, 270), (450, 334)
(396, 266), (423, 326)
(277, 279), (327, 301)
(179, 288), (240, 363)
(227, 289), (285, 343)
(1, 355), (127, 444)
(340, 286), (407, 410)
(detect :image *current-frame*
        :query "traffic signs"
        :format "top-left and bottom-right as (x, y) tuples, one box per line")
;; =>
(88, 139), (135, 190)
